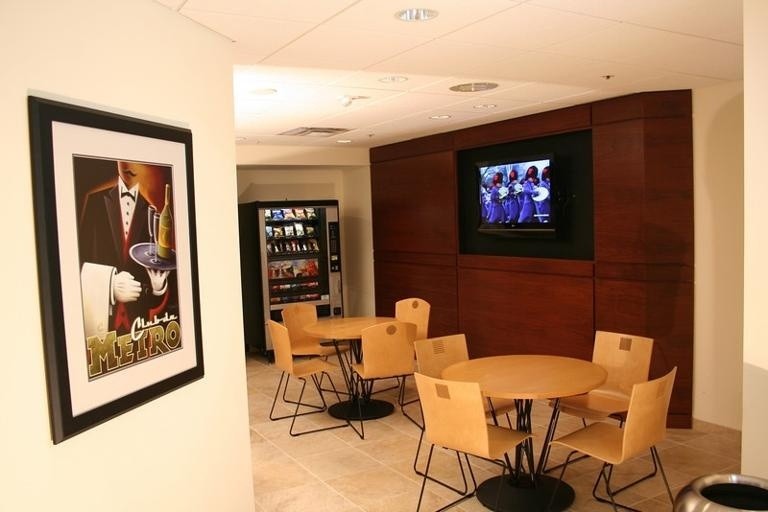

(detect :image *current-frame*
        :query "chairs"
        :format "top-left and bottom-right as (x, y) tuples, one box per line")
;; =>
(542, 330), (655, 473)
(414, 333), (525, 496)
(281, 303), (354, 408)
(366, 297), (431, 405)
(548, 365), (677, 512)
(267, 319), (349, 436)
(347, 321), (425, 439)
(413, 371), (537, 511)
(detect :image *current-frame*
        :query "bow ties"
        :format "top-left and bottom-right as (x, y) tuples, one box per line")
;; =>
(121, 192), (136, 202)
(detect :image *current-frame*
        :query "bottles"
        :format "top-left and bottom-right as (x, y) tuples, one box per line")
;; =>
(157, 182), (174, 261)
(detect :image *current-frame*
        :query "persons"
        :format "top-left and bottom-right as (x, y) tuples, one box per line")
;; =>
(473, 164), (550, 223)
(73, 161), (171, 376)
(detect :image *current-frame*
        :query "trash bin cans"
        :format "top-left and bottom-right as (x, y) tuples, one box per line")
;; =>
(674, 473), (768, 512)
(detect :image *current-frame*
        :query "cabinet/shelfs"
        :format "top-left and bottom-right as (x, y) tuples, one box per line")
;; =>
(28, 96), (205, 445)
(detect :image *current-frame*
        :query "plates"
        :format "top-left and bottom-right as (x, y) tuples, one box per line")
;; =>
(129, 242), (176, 271)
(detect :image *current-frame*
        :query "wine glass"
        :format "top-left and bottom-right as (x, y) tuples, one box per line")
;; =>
(145, 205), (161, 265)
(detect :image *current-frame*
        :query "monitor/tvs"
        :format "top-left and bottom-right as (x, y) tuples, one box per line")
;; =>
(474, 152), (556, 234)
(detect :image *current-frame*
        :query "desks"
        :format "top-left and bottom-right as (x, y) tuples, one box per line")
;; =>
(442, 355), (608, 512)
(303, 317), (398, 420)
